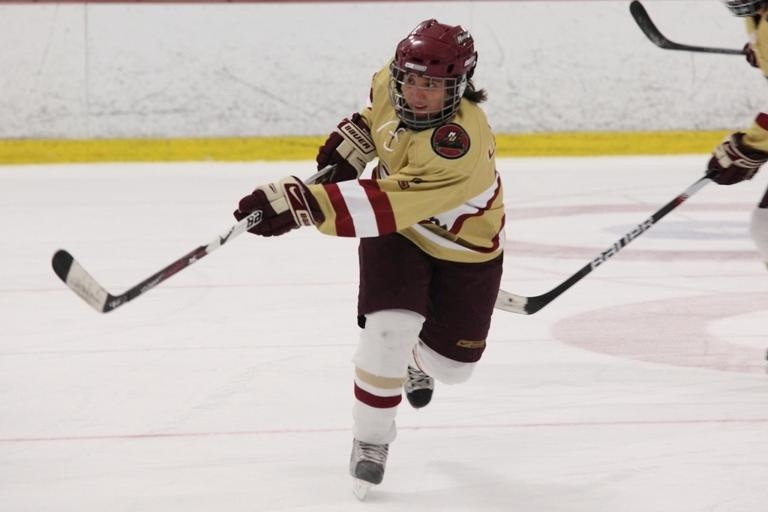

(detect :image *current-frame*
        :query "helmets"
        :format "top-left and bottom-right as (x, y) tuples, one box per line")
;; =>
(388, 19), (478, 131)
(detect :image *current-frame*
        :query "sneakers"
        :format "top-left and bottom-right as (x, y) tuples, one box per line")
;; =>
(349, 438), (388, 484)
(404, 366), (433, 408)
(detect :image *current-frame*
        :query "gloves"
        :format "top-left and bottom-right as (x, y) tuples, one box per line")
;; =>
(234, 175), (325, 237)
(707, 131), (768, 185)
(317, 111), (377, 185)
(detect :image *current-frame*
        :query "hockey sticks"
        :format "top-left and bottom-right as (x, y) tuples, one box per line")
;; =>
(495, 169), (717, 317)
(53, 166), (334, 313)
(631, 0), (748, 54)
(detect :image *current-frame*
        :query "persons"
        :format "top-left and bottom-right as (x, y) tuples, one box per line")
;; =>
(742, 44), (758, 68)
(705, 0), (768, 365)
(235, 18), (506, 486)
